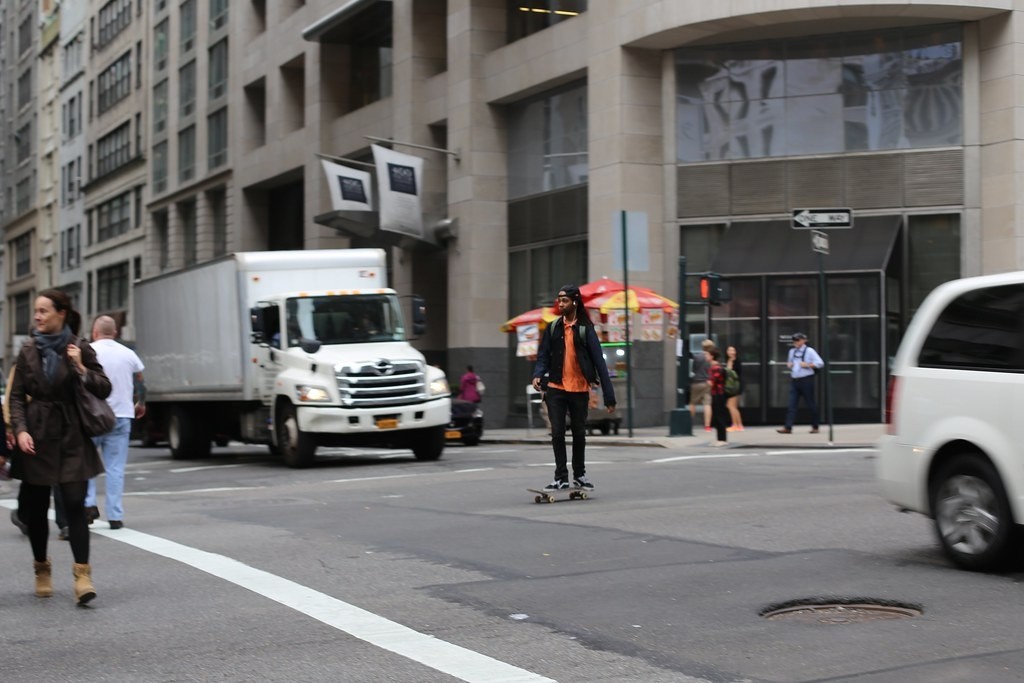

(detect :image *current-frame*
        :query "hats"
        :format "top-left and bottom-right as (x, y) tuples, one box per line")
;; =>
(791, 332), (807, 342)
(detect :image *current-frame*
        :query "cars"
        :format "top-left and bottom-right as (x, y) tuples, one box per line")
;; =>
(446, 399), (484, 445)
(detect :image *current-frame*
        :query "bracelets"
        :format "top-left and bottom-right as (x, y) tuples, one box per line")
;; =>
(806, 364), (809, 368)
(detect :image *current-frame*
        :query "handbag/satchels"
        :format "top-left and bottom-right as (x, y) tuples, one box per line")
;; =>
(63, 338), (116, 434)
(476, 376), (485, 395)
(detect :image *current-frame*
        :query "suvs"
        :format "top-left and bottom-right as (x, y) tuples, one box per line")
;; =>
(874, 272), (1024, 571)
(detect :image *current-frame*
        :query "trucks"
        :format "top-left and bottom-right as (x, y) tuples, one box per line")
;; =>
(133, 248), (451, 468)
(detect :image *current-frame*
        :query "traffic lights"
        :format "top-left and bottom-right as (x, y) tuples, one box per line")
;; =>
(701, 275), (718, 306)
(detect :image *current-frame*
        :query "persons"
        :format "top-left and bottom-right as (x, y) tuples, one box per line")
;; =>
(776, 333), (825, 433)
(532, 285), (617, 492)
(690, 339), (745, 431)
(272, 312), (302, 345)
(8, 289), (112, 603)
(460, 365), (481, 403)
(0, 311), (145, 539)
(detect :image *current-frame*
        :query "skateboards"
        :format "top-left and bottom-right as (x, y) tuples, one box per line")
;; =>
(527, 486), (594, 503)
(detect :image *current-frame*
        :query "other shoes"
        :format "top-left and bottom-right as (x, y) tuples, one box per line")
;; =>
(10, 511), (28, 534)
(85, 505), (99, 518)
(811, 429), (818, 433)
(703, 425), (712, 431)
(776, 428), (790, 434)
(59, 526), (70, 540)
(711, 440), (728, 446)
(108, 518), (123, 530)
(726, 425), (744, 432)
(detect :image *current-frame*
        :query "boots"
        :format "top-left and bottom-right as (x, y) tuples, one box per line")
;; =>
(71, 561), (97, 605)
(34, 557), (53, 597)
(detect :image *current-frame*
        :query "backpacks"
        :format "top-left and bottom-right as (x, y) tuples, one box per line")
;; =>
(716, 367), (741, 396)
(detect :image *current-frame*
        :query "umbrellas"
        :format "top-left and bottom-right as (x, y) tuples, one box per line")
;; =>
(500, 276), (680, 333)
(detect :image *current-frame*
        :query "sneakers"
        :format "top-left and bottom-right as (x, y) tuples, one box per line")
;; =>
(543, 479), (569, 492)
(573, 475), (593, 491)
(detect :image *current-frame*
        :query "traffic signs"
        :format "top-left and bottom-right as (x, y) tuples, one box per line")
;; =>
(811, 230), (830, 254)
(792, 207), (854, 229)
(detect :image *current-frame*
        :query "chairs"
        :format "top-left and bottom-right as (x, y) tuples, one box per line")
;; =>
(332, 311), (354, 339)
(314, 312), (334, 342)
(525, 384), (542, 428)
